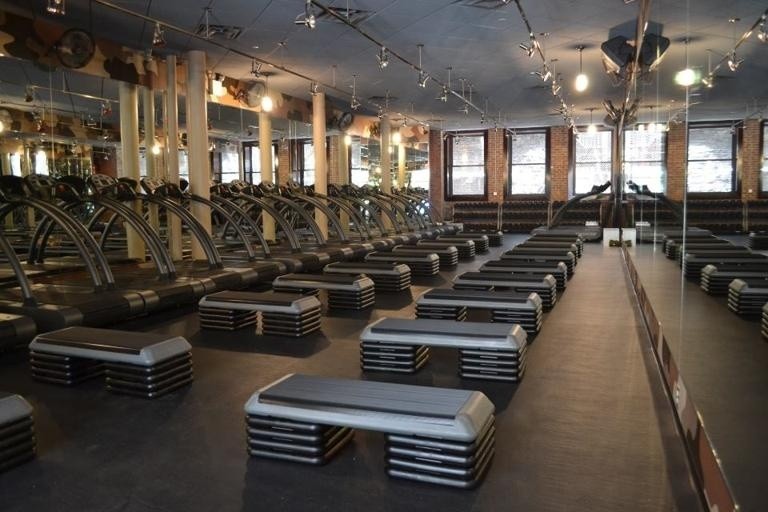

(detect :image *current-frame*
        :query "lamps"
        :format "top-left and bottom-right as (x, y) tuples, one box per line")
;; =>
(25, 0), (583, 142)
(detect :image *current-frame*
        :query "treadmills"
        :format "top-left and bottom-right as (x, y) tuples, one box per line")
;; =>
(0, 311), (38, 351)
(1, 173), (145, 332)
(627, 180), (716, 243)
(1, 172), (466, 284)
(18, 173), (207, 318)
(529, 182), (611, 241)
(169, 172), (287, 289)
(95, 172), (251, 297)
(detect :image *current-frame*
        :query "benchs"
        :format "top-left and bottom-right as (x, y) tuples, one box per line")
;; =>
(662, 232), (767, 341)
(0, 390), (38, 472)
(198, 289), (322, 339)
(272, 272), (376, 311)
(455, 230), (504, 246)
(513, 232), (585, 266)
(28, 325), (194, 400)
(364, 251), (440, 276)
(452, 271), (557, 310)
(414, 287), (544, 335)
(322, 262), (412, 293)
(478, 260), (567, 290)
(358, 317), (528, 383)
(436, 234), (489, 252)
(243, 372), (497, 493)
(392, 244), (459, 266)
(418, 239), (476, 258)
(500, 248), (575, 276)
